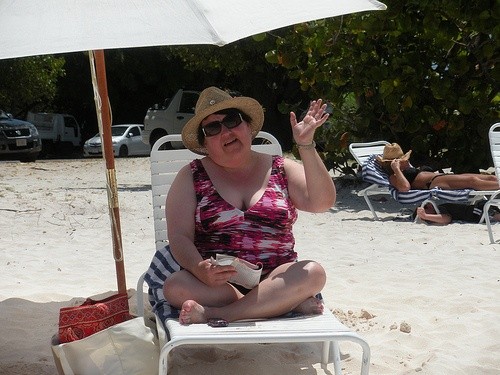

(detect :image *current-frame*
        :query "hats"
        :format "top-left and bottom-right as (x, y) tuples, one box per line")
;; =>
(182, 86), (265, 157)
(378, 142), (412, 163)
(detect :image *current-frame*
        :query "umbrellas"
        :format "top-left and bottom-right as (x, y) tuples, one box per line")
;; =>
(0, 0), (387, 291)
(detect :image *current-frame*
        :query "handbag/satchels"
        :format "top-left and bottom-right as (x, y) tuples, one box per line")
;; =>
(51, 315), (174, 375)
(58, 293), (129, 346)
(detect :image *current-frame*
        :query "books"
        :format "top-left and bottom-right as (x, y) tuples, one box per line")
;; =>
(211, 253), (263, 290)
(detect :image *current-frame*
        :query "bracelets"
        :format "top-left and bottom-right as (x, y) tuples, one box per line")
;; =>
(295, 141), (316, 150)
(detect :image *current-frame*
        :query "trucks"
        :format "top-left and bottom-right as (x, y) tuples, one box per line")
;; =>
(26, 112), (80, 152)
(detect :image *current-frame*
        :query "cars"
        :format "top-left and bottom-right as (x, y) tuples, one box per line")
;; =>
(0, 110), (42, 162)
(82, 124), (151, 156)
(141, 90), (202, 150)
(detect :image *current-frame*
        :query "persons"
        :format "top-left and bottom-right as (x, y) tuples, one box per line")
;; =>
(164, 86), (336, 325)
(379, 143), (500, 192)
(413, 201), (500, 225)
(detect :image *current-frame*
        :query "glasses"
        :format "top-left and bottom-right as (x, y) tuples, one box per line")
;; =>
(199, 112), (244, 137)
